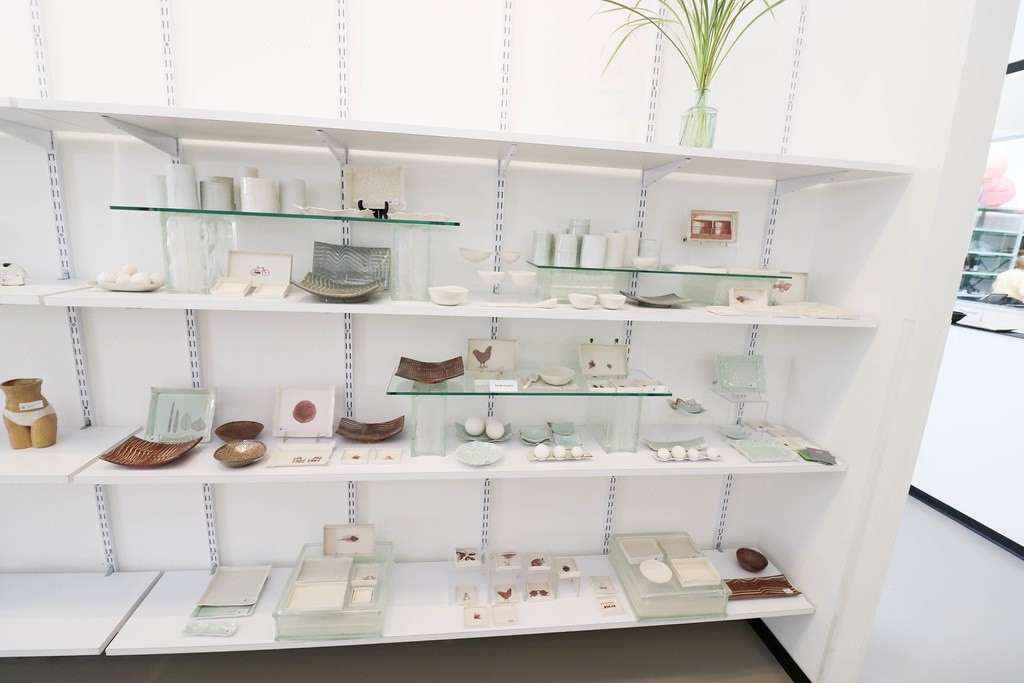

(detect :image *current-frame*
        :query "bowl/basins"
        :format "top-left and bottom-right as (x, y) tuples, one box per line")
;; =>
(213, 438), (266, 468)
(568, 292), (595, 309)
(538, 365), (575, 386)
(737, 547), (769, 572)
(600, 294), (626, 309)
(428, 285), (468, 307)
(461, 247), (536, 289)
(216, 421), (264, 443)
(630, 257), (654, 269)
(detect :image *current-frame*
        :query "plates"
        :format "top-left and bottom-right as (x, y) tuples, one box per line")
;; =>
(666, 397), (707, 417)
(265, 441), (335, 467)
(343, 162), (405, 214)
(395, 356), (463, 384)
(725, 573), (799, 601)
(339, 446), (371, 464)
(622, 289), (691, 307)
(99, 282), (163, 292)
(521, 420), (593, 462)
(670, 263), (849, 320)
(454, 419), (515, 443)
(335, 415), (406, 442)
(455, 440), (505, 465)
(579, 342), (665, 393)
(713, 353), (766, 395)
(532, 377), (579, 389)
(373, 448), (403, 462)
(720, 420), (808, 464)
(210, 240), (392, 303)
(99, 435), (203, 468)
(270, 385), (337, 440)
(181, 522), (725, 629)
(643, 436), (721, 461)
(145, 384), (216, 444)
(464, 337), (518, 373)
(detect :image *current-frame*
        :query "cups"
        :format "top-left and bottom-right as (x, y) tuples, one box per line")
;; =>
(641, 237), (661, 270)
(530, 217), (626, 268)
(136, 161), (306, 216)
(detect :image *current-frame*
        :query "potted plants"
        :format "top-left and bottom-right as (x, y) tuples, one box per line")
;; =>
(588, 0), (788, 150)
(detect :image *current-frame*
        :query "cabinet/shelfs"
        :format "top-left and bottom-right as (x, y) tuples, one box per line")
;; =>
(0, 90), (914, 660)
(956, 208), (1024, 301)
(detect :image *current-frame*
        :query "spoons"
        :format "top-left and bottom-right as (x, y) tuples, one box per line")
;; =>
(521, 373), (539, 389)
